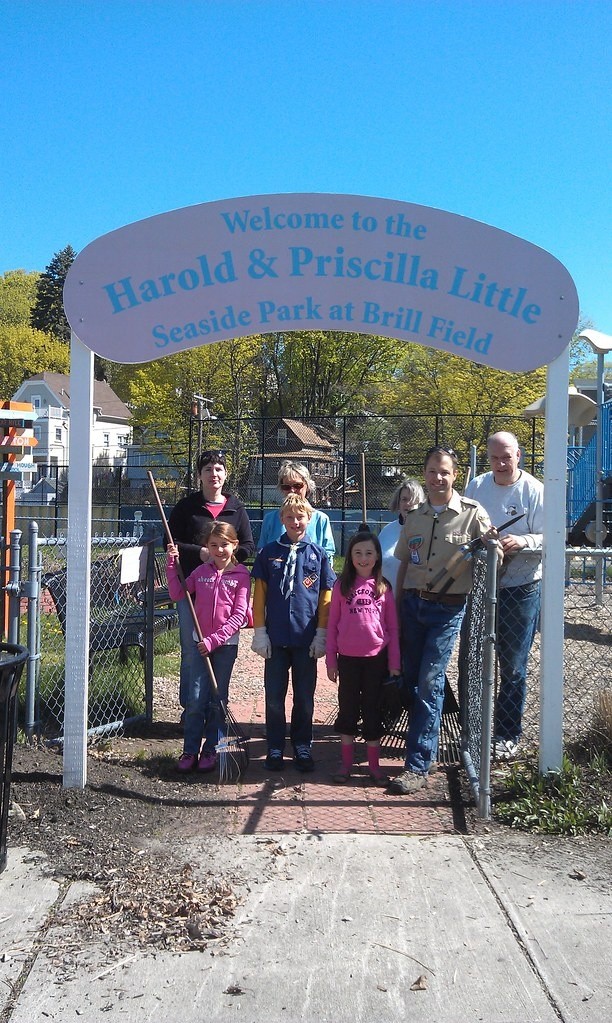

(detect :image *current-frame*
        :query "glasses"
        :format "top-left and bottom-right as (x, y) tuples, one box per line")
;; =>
(280, 481), (306, 491)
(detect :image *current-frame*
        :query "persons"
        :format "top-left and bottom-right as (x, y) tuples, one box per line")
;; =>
(466, 431), (543, 756)
(163, 450), (255, 734)
(249, 493), (338, 771)
(257, 461), (336, 570)
(325, 532), (401, 787)
(166, 521), (251, 774)
(390, 446), (503, 794)
(378, 480), (424, 599)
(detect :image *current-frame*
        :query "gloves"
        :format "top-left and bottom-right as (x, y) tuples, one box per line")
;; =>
(309, 627), (327, 659)
(251, 626), (272, 659)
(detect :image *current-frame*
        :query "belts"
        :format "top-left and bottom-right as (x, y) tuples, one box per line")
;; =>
(406, 589), (467, 605)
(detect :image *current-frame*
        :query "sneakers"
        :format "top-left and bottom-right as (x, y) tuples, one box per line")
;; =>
(292, 744), (315, 772)
(176, 752), (198, 771)
(392, 769), (427, 794)
(264, 746), (284, 772)
(198, 752), (218, 774)
(488, 737), (518, 759)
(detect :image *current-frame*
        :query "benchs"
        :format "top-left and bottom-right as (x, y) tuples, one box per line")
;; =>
(38, 552), (180, 685)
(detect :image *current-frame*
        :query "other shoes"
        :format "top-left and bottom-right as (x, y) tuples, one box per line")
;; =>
(365, 767), (390, 787)
(334, 766), (352, 783)
